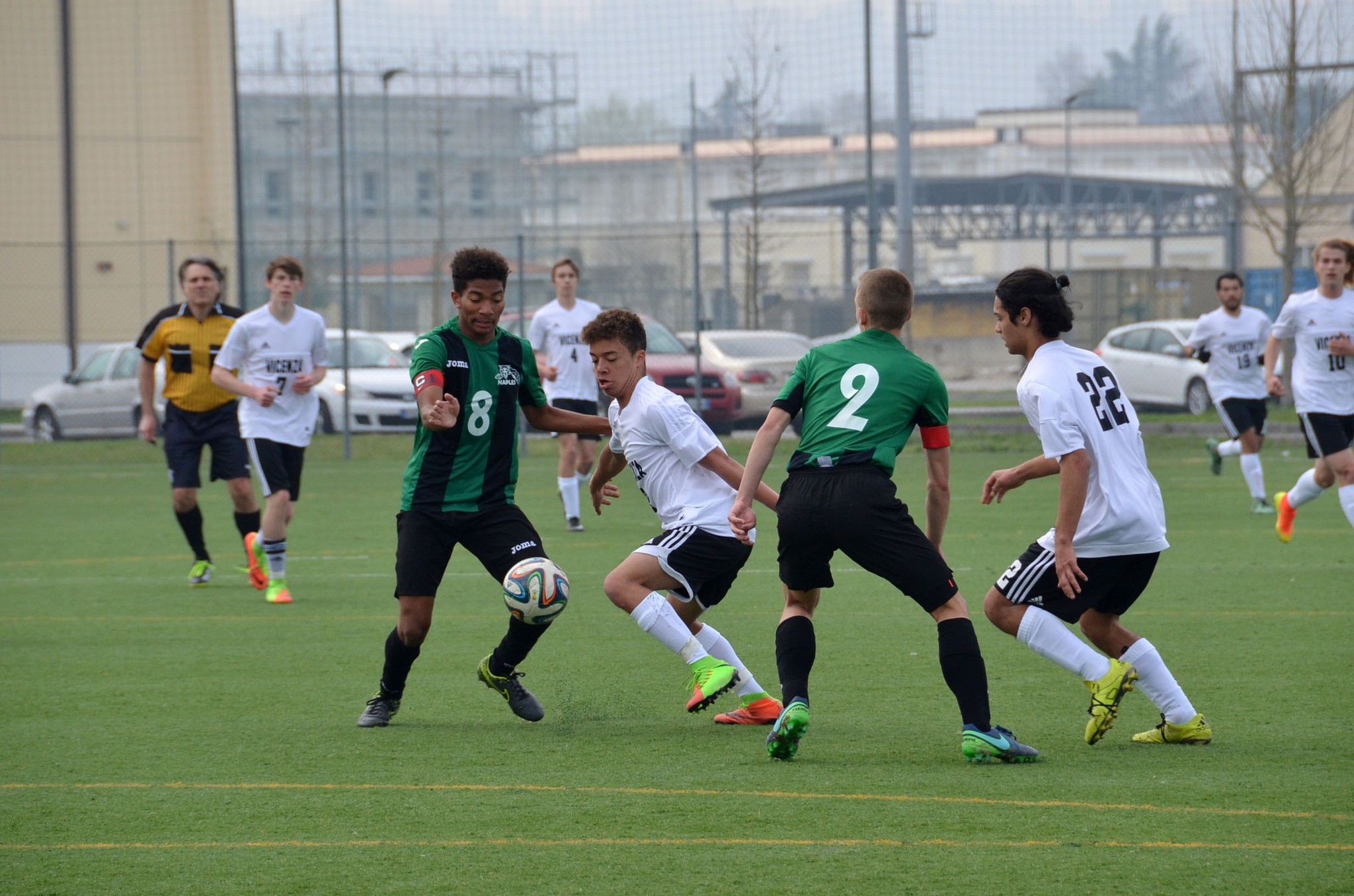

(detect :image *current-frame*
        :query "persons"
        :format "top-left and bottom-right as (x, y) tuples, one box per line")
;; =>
(211, 255), (328, 605)
(728, 268), (1041, 764)
(983, 268), (1213, 744)
(584, 309), (779, 725)
(135, 257), (265, 586)
(1184, 272), (1285, 514)
(357, 249), (612, 727)
(527, 258), (604, 532)
(1263, 241), (1354, 544)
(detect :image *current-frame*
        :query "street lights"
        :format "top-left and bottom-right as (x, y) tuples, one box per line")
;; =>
(1064, 87), (1093, 290)
(379, 68), (412, 330)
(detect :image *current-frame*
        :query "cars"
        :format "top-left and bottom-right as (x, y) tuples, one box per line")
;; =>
(675, 328), (820, 423)
(18, 341), (171, 442)
(1091, 319), (1214, 416)
(631, 312), (743, 433)
(312, 329), (423, 434)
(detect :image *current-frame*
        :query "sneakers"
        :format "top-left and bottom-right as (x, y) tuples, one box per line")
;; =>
(357, 692), (401, 725)
(187, 560), (216, 587)
(765, 697), (810, 761)
(243, 532), (270, 590)
(1205, 438), (1222, 475)
(713, 696), (783, 724)
(1085, 656), (1139, 744)
(1251, 498), (1275, 514)
(1272, 491), (1299, 543)
(567, 516), (584, 531)
(960, 723), (1039, 763)
(263, 578), (293, 603)
(1133, 713), (1212, 744)
(680, 659), (741, 713)
(477, 653), (545, 722)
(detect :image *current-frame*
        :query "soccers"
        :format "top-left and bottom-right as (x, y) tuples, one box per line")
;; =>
(503, 557), (570, 625)
